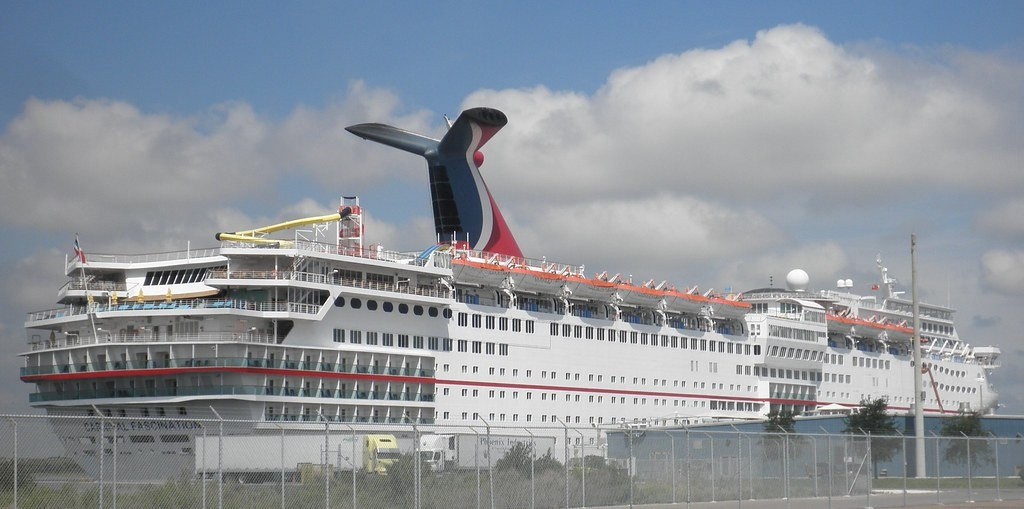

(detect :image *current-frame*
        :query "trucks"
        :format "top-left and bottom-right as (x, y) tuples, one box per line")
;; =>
(363, 433), (400, 476)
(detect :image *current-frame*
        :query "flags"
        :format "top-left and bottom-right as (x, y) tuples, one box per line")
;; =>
(137, 288), (144, 304)
(111, 291), (117, 304)
(166, 288), (172, 303)
(88, 294), (94, 305)
(72, 238), (86, 264)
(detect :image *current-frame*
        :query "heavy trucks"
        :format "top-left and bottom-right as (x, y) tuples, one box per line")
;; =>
(416, 432), (557, 476)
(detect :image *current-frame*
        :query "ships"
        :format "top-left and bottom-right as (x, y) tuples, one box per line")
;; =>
(15, 101), (1003, 486)
